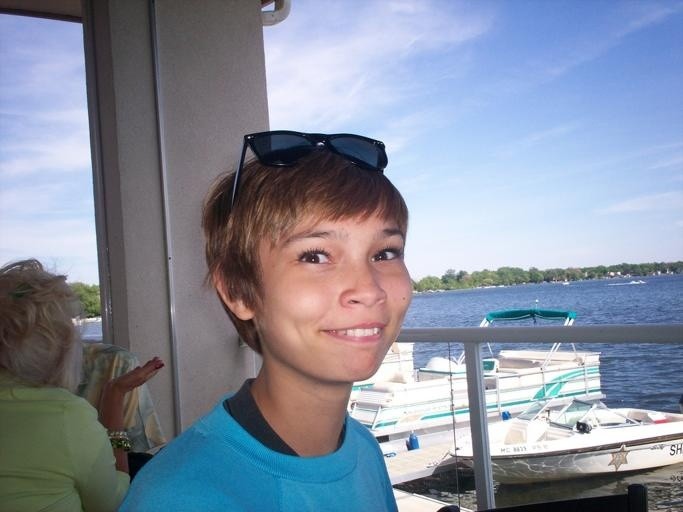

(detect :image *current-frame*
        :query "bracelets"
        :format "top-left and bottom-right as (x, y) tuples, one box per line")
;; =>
(110, 441), (132, 451)
(107, 436), (131, 440)
(105, 429), (131, 435)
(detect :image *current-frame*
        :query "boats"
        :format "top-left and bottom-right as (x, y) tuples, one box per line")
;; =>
(348, 300), (607, 485)
(629, 279), (648, 284)
(447, 394), (683, 484)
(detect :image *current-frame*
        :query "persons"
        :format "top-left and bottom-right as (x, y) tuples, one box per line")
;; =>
(116, 129), (416, 512)
(0, 258), (165, 511)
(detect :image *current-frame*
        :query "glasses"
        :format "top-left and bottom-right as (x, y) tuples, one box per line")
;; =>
(227, 131), (388, 215)
